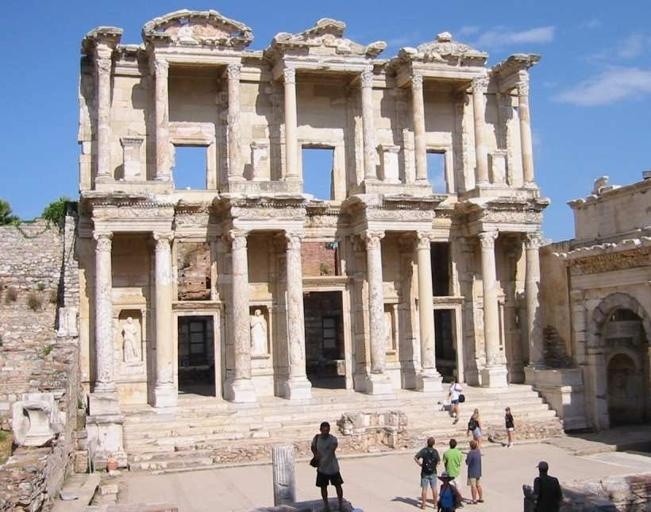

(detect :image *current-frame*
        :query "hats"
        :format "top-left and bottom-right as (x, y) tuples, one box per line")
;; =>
(437, 472), (454, 481)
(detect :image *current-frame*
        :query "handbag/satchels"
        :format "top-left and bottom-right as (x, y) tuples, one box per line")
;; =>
(309, 456), (319, 466)
(458, 393), (464, 402)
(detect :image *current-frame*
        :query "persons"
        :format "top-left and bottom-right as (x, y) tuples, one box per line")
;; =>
(413, 437), (442, 510)
(122, 316), (141, 363)
(442, 438), (463, 492)
(526, 460), (564, 512)
(249, 307), (270, 358)
(503, 406), (517, 448)
(447, 376), (465, 419)
(437, 472), (464, 512)
(466, 408), (483, 449)
(310, 421), (350, 512)
(465, 440), (485, 505)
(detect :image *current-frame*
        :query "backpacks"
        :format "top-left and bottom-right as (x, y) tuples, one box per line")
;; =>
(422, 447), (437, 475)
(468, 419), (476, 430)
